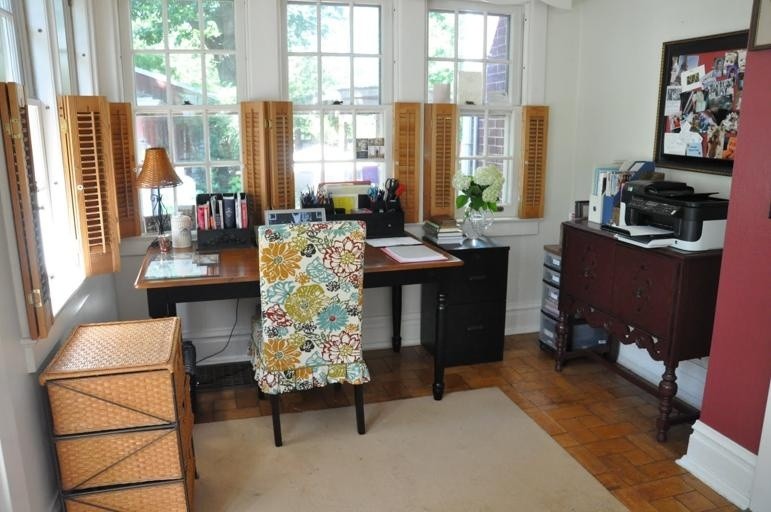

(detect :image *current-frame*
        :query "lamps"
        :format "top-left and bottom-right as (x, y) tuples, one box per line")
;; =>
(135, 145), (183, 249)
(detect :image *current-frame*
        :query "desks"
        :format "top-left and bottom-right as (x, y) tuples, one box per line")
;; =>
(556, 218), (724, 441)
(134, 231), (465, 400)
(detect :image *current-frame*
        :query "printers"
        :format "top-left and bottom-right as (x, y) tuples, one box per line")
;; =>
(599, 180), (729, 251)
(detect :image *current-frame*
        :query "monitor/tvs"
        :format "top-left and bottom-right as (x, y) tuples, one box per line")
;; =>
(264, 207), (326, 225)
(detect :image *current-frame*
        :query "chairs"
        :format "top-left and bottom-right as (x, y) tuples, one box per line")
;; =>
(247, 218), (371, 446)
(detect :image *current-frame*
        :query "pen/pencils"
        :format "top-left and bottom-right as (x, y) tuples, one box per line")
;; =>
(300, 184), (333, 206)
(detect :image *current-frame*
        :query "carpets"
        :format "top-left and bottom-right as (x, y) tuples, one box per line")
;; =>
(191, 383), (630, 512)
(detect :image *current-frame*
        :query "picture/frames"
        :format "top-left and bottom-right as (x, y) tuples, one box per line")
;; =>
(653, 30), (749, 177)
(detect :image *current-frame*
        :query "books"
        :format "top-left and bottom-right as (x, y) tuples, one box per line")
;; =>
(198, 192), (249, 230)
(422, 213), (464, 246)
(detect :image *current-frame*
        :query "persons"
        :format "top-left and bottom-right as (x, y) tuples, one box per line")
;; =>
(667, 49), (747, 161)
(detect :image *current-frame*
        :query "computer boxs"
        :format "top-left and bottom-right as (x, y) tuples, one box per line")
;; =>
(182, 343), (200, 413)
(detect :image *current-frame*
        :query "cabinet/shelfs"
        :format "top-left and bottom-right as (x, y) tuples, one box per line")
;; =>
(421, 234), (511, 369)
(539, 244), (612, 366)
(38, 316), (199, 512)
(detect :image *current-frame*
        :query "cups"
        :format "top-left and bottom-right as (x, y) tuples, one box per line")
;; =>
(158, 234), (171, 252)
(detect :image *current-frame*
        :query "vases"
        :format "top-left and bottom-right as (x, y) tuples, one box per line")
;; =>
(462, 202), (497, 241)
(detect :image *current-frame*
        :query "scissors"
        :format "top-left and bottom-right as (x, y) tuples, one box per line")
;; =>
(368, 178), (406, 203)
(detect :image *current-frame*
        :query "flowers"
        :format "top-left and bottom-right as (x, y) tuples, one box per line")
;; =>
(452, 164), (506, 221)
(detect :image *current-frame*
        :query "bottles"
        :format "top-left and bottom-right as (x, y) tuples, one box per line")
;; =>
(171, 212), (192, 248)
